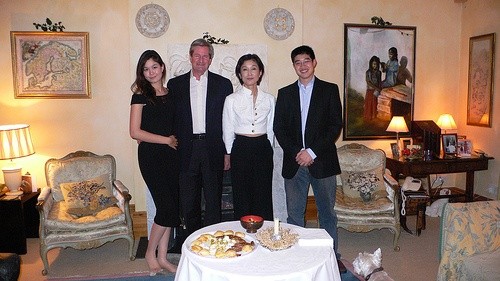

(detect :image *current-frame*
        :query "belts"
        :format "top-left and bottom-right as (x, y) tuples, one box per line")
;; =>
(193, 134), (207, 140)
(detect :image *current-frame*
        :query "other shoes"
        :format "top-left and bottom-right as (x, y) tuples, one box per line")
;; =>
(337, 259), (347, 274)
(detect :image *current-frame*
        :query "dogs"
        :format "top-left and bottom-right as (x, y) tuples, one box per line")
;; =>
(425, 189), (451, 217)
(353, 248), (395, 281)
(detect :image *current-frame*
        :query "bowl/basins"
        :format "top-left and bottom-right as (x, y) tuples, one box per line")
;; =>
(240, 215), (264, 232)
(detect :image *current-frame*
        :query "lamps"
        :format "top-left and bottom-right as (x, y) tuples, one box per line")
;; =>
(437, 114), (458, 133)
(0, 124), (35, 191)
(386, 115), (408, 157)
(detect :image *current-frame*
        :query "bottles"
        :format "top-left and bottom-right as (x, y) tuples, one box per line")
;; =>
(460, 139), (472, 153)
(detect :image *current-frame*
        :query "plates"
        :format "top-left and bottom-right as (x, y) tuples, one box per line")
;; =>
(186, 231), (256, 258)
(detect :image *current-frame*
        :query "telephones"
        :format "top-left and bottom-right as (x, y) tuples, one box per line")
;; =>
(402, 177), (421, 191)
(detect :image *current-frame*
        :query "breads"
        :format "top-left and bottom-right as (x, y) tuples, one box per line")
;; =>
(190, 230), (253, 258)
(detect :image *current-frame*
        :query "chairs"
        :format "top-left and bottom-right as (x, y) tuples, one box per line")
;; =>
(317, 143), (403, 252)
(35, 150), (134, 275)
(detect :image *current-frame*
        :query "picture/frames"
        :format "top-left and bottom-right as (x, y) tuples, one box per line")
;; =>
(343, 22), (416, 141)
(466, 32), (497, 128)
(442, 133), (458, 155)
(400, 138), (413, 158)
(9, 31), (93, 100)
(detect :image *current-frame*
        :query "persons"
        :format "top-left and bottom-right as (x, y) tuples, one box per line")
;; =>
(130, 50), (179, 275)
(222, 54), (276, 223)
(273, 45), (347, 274)
(167, 39), (234, 252)
(446, 137), (455, 153)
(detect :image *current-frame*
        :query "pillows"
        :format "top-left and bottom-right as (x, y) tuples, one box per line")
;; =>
(340, 167), (389, 201)
(60, 173), (118, 219)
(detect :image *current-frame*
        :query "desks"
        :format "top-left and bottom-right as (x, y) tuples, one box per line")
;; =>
(174, 221), (342, 281)
(385, 154), (496, 202)
(0, 192), (40, 254)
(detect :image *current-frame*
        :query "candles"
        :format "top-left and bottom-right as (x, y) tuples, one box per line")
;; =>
(274, 218), (279, 238)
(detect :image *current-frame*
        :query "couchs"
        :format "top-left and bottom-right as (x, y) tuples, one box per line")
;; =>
(437, 199), (500, 281)
